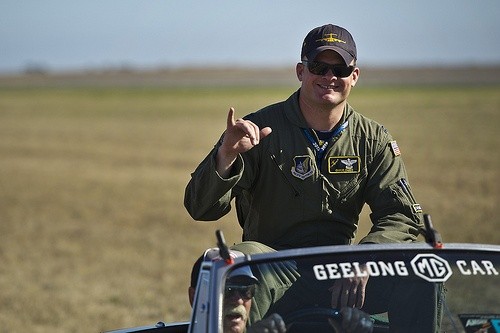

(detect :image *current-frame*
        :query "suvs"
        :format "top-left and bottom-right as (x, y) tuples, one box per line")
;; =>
(99, 213), (500, 333)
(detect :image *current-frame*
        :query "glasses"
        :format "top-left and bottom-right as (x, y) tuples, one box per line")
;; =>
(224, 284), (256, 300)
(301, 59), (355, 77)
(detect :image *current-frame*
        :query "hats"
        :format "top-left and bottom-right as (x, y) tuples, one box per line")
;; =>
(301, 23), (357, 67)
(190, 247), (259, 286)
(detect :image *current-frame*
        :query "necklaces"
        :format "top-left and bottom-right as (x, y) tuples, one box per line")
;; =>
(303, 120), (349, 169)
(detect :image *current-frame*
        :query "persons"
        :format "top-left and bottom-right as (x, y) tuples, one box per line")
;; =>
(188, 247), (373, 333)
(184, 23), (447, 333)
